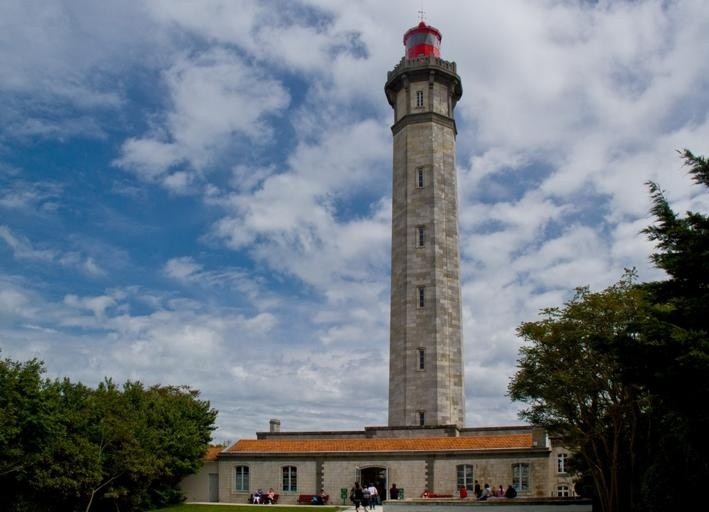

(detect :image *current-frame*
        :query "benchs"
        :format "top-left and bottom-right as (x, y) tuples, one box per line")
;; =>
(249, 493), (279, 504)
(297, 493), (328, 504)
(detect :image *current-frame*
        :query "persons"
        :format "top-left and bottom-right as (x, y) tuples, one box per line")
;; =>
(460, 484), (467, 498)
(252, 489), (263, 504)
(474, 479), (517, 499)
(349, 480), (384, 512)
(390, 483), (399, 499)
(320, 490), (326, 504)
(263, 487), (275, 504)
